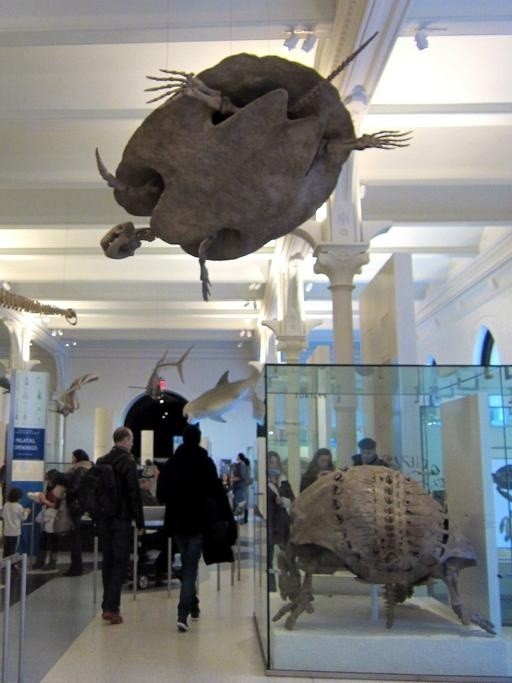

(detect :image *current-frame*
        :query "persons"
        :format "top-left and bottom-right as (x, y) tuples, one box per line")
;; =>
(262, 451), (298, 595)
(347, 431), (388, 467)
(295, 445), (331, 497)
(89, 426), (148, 622)
(0, 436), (254, 572)
(156, 424), (240, 629)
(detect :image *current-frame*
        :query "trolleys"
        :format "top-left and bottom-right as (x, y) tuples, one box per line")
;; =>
(122, 522), (183, 590)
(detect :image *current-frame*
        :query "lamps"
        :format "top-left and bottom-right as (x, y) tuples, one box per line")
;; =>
(413, 22), (444, 55)
(276, 23), (321, 58)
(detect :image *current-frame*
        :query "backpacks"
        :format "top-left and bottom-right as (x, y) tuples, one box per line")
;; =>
(86, 448), (127, 519)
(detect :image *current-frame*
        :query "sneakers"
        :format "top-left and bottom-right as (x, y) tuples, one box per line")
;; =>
(177, 618), (190, 631)
(103, 611), (123, 624)
(32, 562), (56, 570)
(190, 613), (198, 617)
